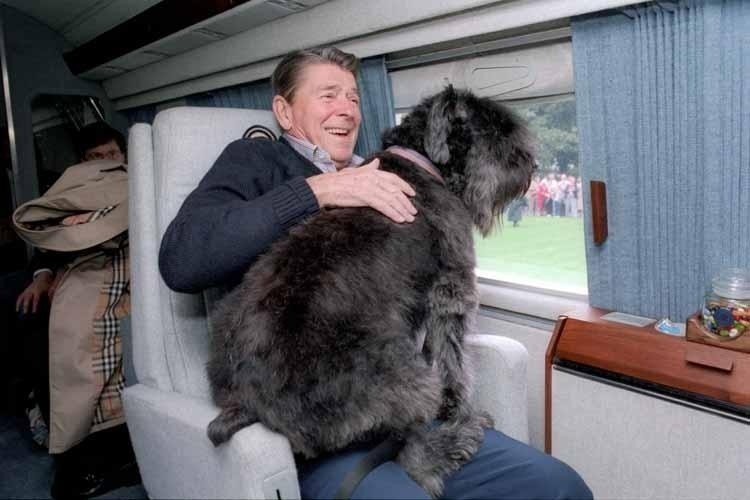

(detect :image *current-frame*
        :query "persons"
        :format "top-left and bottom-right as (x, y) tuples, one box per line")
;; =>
(504, 167), (583, 229)
(159, 45), (597, 499)
(13, 122), (142, 430)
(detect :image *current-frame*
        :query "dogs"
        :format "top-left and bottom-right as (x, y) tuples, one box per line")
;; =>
(199, 79), (542, 499)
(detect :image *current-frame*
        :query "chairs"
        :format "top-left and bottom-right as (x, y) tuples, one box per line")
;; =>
(119, 101), (536, 500)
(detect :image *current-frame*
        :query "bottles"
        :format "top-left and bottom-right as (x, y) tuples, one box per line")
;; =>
(699, 268), (750, 335)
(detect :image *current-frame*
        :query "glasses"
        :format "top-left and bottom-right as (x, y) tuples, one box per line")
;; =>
(86, 150), (123, 161)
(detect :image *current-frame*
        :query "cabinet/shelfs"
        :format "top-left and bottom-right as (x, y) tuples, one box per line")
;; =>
(540, 306), (749, 500)
